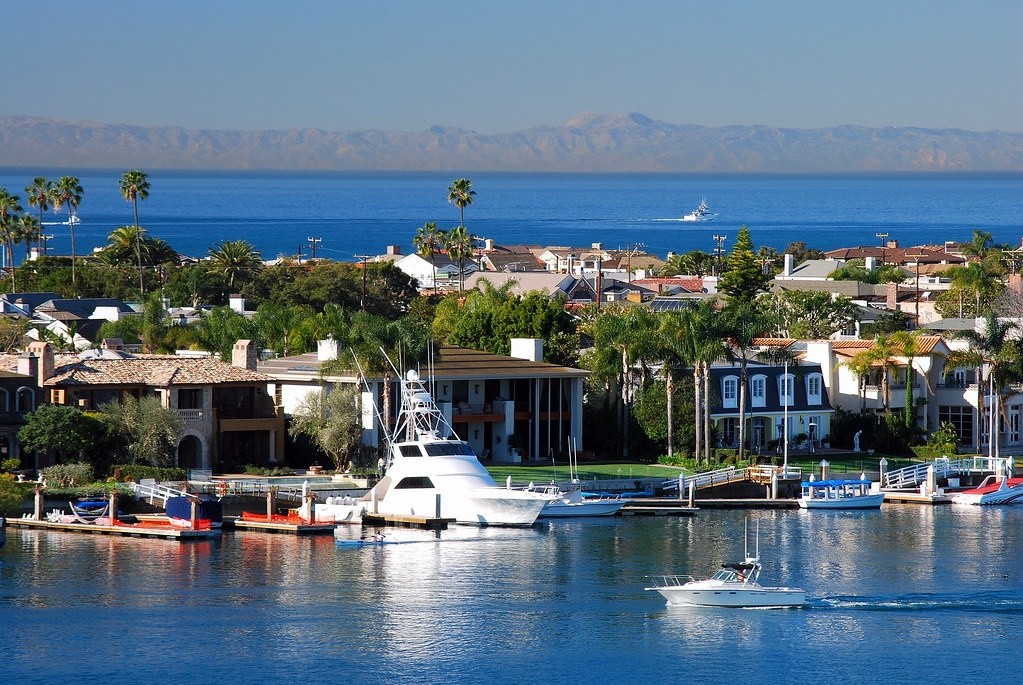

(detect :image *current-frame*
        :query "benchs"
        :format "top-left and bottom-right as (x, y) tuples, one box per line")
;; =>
(571, 451), (593, 462)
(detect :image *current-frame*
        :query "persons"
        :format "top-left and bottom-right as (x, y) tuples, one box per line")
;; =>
(853, 429), (862, 450)
(377, 457), (386, 476)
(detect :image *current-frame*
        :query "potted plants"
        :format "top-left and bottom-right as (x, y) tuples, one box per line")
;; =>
(821, 434), (832, 449)
(795, 433), (808, 450)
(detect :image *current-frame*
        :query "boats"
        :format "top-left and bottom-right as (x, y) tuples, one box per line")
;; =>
(348, 343), (563, 527)
(528, 433), (625, 518)
(642, 516), (807, 606)
(798, 480), (886, 510)
(951, 475), (1023, 505)
(684, 200), (719, 221)
